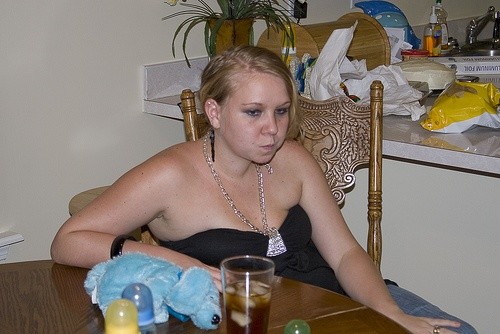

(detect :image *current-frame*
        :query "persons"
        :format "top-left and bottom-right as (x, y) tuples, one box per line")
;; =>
(50, 45), (478, 334)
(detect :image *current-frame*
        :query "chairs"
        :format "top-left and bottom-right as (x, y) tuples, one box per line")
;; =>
(181, 78), (384, 271)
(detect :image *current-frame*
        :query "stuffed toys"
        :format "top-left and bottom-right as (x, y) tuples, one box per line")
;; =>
(84, 251), (222, 330)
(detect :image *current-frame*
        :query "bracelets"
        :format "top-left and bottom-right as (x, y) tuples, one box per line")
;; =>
(110, 234), (137, 259)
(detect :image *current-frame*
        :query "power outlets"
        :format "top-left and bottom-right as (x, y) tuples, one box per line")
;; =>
(288, 0), (306, 16)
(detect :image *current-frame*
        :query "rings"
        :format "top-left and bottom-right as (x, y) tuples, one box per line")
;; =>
(433, 326), (440, 334)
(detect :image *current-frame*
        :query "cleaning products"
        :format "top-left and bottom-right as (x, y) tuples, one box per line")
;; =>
(434, 0), (449, 50)
(423, 5), (442, 57)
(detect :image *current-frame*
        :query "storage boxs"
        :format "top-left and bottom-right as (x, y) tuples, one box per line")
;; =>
(428, 56), (500, 75)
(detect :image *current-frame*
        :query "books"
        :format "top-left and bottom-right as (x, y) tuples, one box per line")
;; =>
(0, 231), (24, 261)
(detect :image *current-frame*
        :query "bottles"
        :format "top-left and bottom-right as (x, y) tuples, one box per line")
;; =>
(104, 299), (142, 334)
(284, 319), (311, 334)
(122, 283), (157, 334)
(435, 0), (449, 49)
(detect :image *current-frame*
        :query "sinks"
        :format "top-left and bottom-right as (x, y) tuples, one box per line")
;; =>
(462, 40), (500, 56)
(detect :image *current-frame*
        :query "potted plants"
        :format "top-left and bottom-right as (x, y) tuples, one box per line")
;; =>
(160, 0), (306, 69)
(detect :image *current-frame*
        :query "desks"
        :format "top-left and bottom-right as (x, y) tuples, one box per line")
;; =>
(0, 259), (413, 334)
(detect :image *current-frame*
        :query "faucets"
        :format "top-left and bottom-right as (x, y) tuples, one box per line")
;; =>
(491, 11), (499, 43)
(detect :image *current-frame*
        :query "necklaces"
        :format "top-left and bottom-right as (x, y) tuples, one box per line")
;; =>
(202, 130), (287, 257)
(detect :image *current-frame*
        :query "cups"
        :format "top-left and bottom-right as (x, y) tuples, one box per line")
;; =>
(219, 254), (276, 334)
